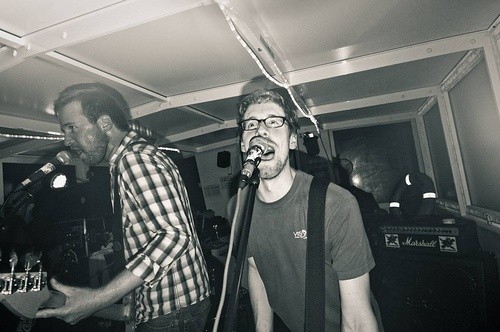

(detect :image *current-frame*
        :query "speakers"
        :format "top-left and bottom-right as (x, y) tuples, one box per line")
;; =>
(368, 248), (500, 332)
(217, 150), (231, 168)
(305, 136), (320, 155)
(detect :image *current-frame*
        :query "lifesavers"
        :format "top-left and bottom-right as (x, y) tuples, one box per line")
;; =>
(389, 172), (437, 216)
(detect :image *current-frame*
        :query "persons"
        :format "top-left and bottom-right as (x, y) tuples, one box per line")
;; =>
(34, 83), (213, 332)
(226, 89), (383, 332)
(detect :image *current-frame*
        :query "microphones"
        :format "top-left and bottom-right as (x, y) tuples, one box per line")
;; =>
(236, 135), (268, 189)
(15, 151), (72, 192)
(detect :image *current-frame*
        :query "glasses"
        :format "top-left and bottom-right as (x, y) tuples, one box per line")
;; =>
(237, 116), (291, 131)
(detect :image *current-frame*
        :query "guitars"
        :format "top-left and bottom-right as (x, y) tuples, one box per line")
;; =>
(0, 250), (136, 332)
